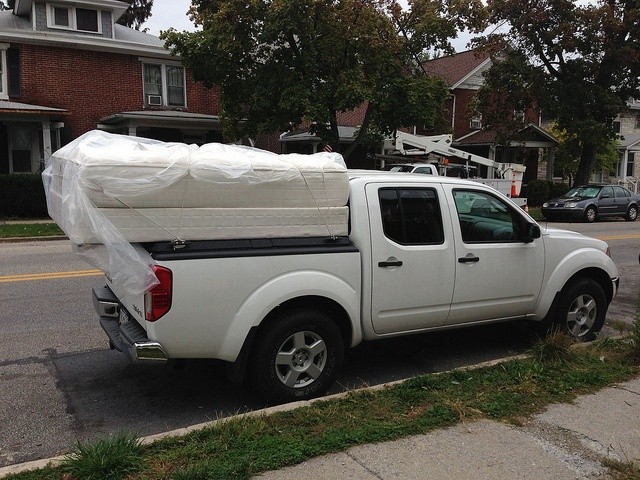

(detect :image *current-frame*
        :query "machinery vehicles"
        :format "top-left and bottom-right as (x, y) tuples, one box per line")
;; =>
(389, 130), (528, 213)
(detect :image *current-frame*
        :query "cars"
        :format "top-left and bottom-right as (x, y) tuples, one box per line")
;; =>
(540, 183), (640, 222)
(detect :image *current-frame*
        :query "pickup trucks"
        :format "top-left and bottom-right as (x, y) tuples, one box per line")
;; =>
(92, 168), (620, 401)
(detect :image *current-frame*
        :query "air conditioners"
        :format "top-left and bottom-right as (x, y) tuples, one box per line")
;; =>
(145, 95), (162, 106)
